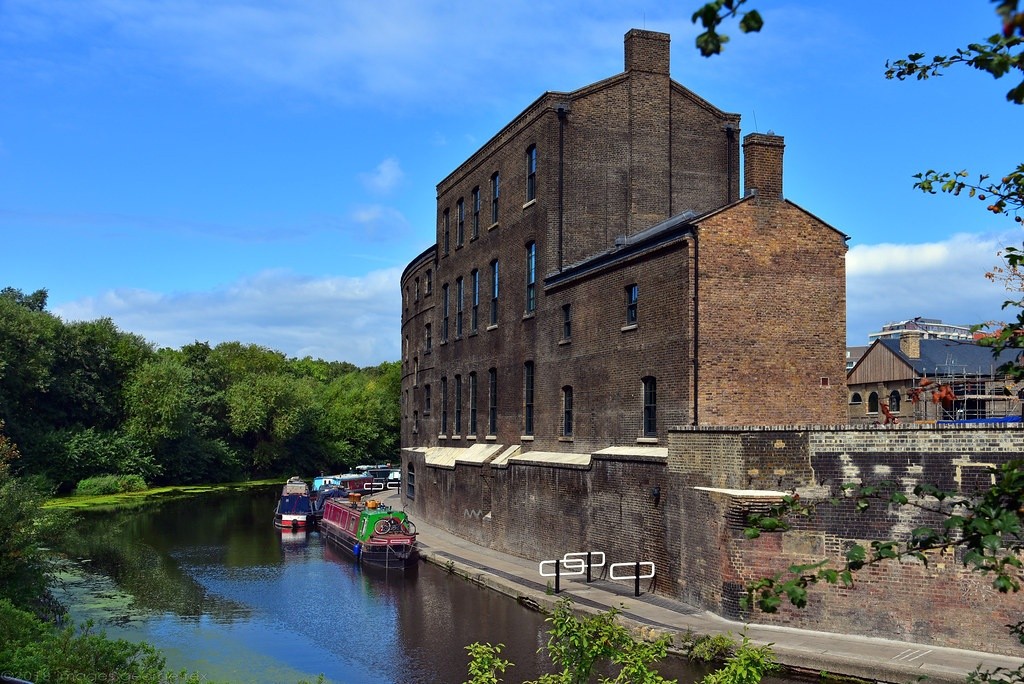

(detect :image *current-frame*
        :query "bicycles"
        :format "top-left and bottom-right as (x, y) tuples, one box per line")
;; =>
(374, 504), (417, 536)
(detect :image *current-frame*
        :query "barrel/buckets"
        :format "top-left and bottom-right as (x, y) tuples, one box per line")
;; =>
(354, 494), (361, 502)
(353, 544), (359, 556)
(349, 493), (354, 502)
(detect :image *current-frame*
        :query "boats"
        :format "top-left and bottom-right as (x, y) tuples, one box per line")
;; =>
(272, 462), (403, 530)
(318, 491), (420, 570)
